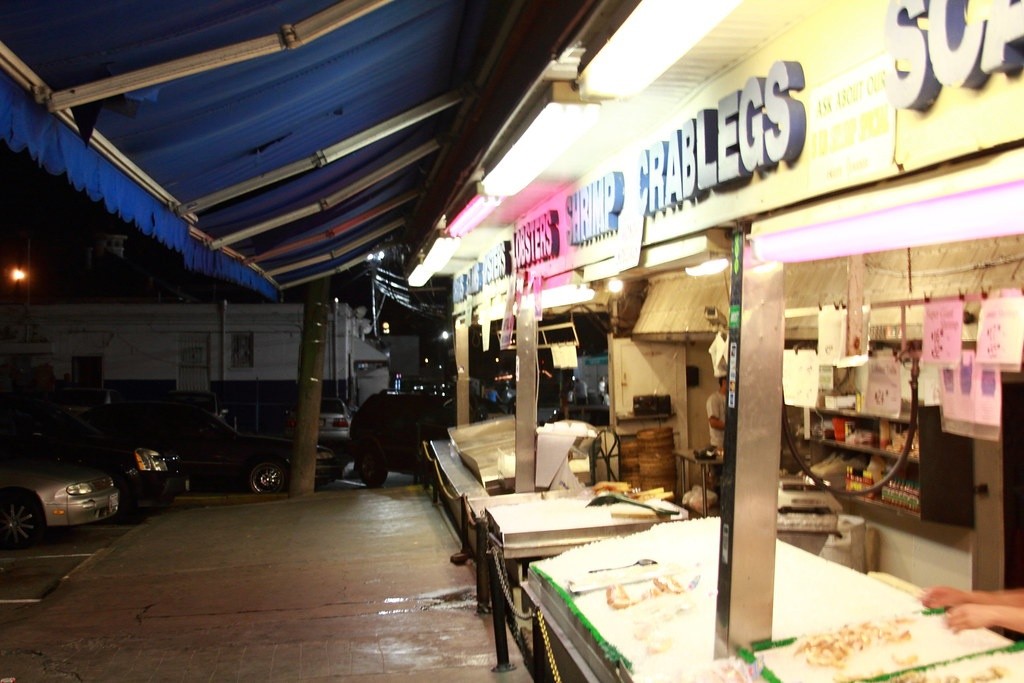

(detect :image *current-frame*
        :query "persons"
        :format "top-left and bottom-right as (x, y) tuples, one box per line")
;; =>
(487, 389), (503, 403)
(563, 375), (609, 406)
(705, 375), (728, 508)
(920, 586), (1024, 635)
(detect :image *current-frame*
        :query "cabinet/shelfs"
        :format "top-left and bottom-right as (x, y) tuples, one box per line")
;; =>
(811, 403), (974, 528)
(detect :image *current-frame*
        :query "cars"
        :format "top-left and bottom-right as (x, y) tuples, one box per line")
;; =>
(0, 440), (118, 551)
(124, 400), (339, 494)
(6, 408), (180, 521)
(280, 394), (355, 445)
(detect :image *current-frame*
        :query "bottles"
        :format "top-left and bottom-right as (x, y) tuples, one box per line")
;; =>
(845, 464), (921, 514)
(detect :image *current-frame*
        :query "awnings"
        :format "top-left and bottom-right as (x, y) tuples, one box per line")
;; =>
(0, 1), (519, 301)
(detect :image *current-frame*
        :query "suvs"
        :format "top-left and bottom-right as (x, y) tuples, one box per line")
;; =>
(346, 390), (488, 488)
